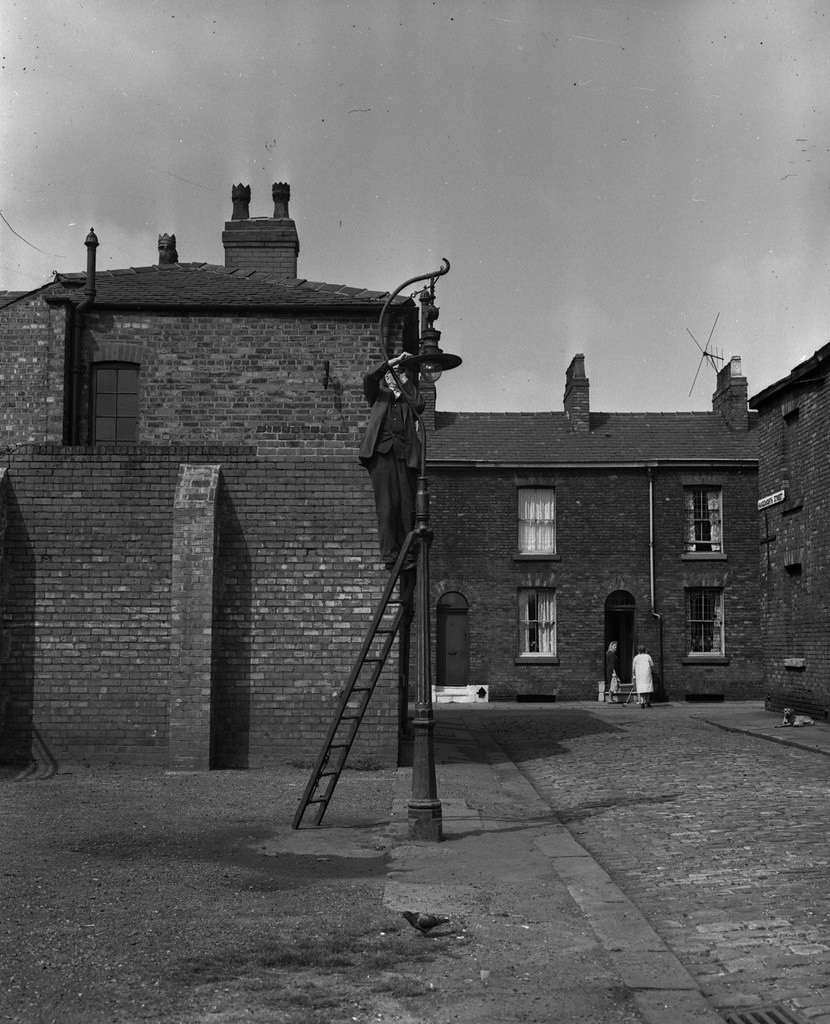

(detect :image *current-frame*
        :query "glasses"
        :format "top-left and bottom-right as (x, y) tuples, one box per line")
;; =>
(388, 369), (404, 374)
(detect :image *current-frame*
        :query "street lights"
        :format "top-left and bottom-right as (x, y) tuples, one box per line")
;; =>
(376, 256), (463, 842)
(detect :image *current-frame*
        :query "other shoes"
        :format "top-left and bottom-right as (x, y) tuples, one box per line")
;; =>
(640, 703), (646, 709)
(385, 561), (417, 571)
(646, 702), (651, 709)
(608, 701), (616, 704)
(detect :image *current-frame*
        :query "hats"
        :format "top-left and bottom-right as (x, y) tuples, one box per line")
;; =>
(381, 359), (407, 376)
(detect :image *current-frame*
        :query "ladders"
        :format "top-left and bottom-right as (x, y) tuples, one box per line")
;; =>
(291, 530), (434, 830)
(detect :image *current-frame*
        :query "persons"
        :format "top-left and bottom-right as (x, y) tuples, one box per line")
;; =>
(605, 640), (620, 704)
(632, 645), (654, 710)
(358, 353), (426, 571)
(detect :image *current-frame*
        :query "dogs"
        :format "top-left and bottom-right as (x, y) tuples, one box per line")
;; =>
(771, 707), (815, 728)
